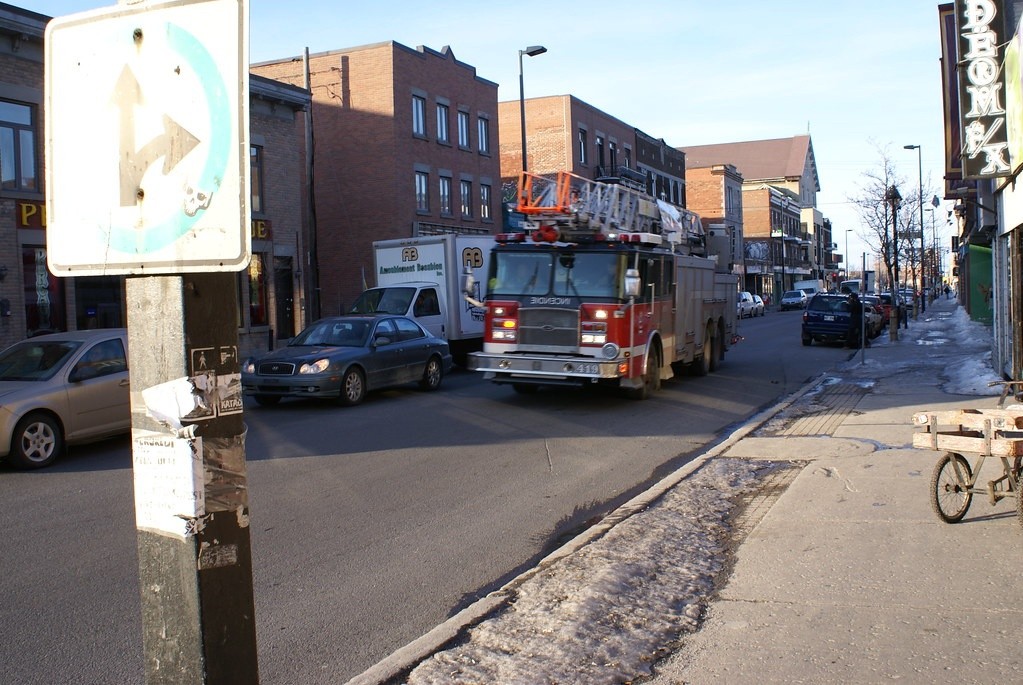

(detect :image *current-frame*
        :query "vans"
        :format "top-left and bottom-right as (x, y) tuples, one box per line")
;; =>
(737, 292), (755, 320)
(840, 280), (862, 295)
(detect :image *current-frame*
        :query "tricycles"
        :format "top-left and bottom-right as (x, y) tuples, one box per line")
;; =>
(930, 378), (1023, 526)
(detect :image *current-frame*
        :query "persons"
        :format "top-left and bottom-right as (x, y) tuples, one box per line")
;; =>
(505, 262), (548, 292)
(944, 286), (950, 300)
(841, 291), (871, 350)
(595, 258), (617, 287)
(897, 306), (904, 329)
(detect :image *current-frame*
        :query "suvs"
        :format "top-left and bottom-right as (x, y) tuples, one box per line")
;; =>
(801, 291), (868, 349)
(781, 290), (808, 312)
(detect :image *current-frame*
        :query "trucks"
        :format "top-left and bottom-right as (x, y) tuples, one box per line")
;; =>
(331, 232), (578, 372)
(794, 279), (823, 305)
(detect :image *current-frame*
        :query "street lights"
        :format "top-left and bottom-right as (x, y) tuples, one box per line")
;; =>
(924, 208), (936, 300)
(780, 194), (790, 292)
(518, 45), (548, 236)
(903, 143), (926, 313)
(845, 229), (852, 280)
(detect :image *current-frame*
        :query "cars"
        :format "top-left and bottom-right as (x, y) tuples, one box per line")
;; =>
(752, 294), (764, 317)
(858, 286), (935, 340)
(1, 326), (130, 470)
(241, 311), (455, 409)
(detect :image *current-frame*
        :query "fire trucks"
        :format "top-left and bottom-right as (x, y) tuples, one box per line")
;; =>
(460, 172), (744, 399)
